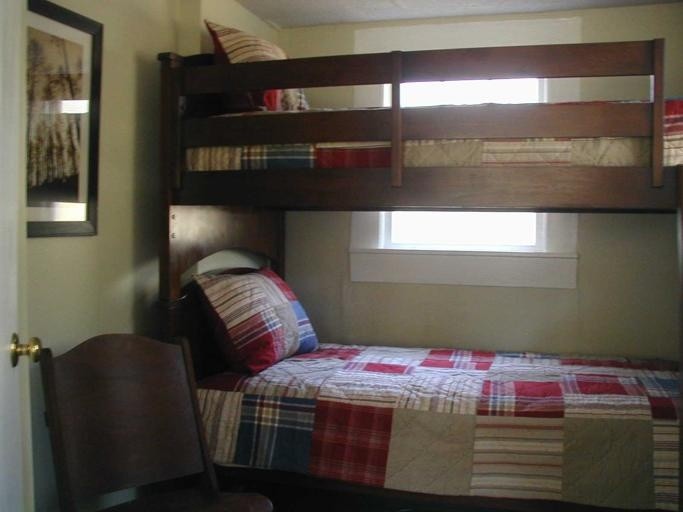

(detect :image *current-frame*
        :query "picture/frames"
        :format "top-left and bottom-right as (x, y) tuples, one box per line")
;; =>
(25, 1), (104, 239)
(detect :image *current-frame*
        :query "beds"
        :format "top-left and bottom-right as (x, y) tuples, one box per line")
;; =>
(157, 38), (681, 215)
(158, 266), (681, 510)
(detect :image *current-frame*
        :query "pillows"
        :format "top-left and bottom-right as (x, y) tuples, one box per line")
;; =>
(204, 20), (310, 111)
(190, 266), (319, 374)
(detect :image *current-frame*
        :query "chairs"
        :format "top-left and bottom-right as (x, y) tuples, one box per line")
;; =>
(37, 333), (274, 511)
